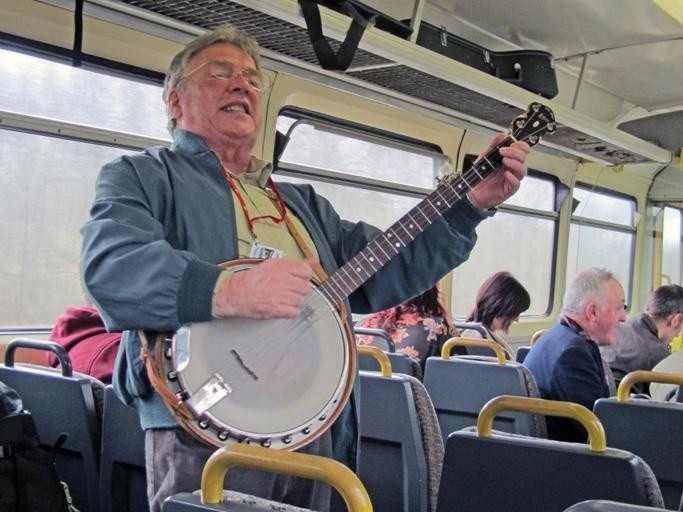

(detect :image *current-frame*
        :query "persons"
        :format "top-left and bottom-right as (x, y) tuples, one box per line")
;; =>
(456, 271), (530, 361)
(521, 266), (627, 445)
(352, 282), (468, 376)
(596, 283), (683, 398)
(74, 18), (534, 512)
(44, 256), (124, 388)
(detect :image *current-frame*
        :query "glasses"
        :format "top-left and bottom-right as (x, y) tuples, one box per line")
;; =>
(172, 57), (274, 97)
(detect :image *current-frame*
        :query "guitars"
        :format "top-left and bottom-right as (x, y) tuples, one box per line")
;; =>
(149, 101), (557, 456)
